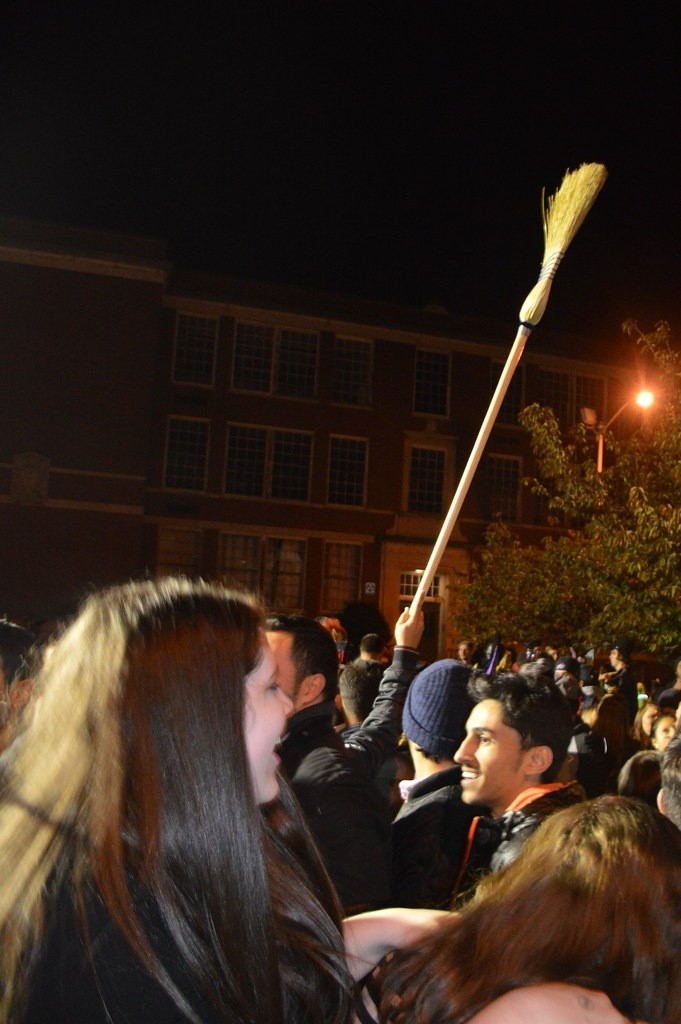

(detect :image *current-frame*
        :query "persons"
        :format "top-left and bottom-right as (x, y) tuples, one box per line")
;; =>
(448, 670), (586, 913)
(390, 657), (478, 909)
(350, 795), (681, 1024)
(0, 575), (632, 1024)
(339, 634), (681, 829)
(264, 613), (390, 916)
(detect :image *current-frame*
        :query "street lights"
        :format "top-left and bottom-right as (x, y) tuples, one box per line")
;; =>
(597, 389), (654, 472)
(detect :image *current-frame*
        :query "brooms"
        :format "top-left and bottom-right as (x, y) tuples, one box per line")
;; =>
(408, 162), (609, 618)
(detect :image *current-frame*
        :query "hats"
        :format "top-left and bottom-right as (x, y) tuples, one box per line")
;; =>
(554, 655), (581, 682)
(402, 658), (479, 760)
